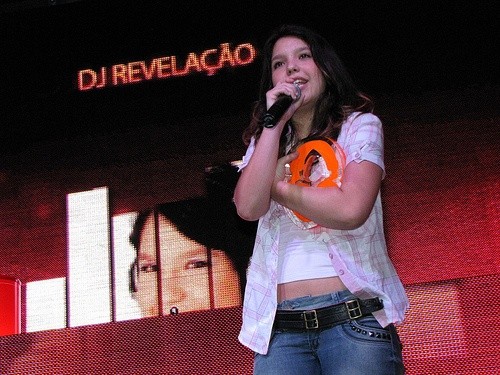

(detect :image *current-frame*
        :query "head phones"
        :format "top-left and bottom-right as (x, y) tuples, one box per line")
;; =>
(282, 134), (347, 230)
(128, 259), (138, 299)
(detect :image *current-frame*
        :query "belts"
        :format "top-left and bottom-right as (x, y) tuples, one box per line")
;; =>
(273, 297), (383, 331)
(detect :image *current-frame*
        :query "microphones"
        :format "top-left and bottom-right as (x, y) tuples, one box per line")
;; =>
(262, 84), (301, 128)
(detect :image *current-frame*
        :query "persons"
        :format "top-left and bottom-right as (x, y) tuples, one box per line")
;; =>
(128, 194), (258, 318)
(234, 24), (410, 375)
